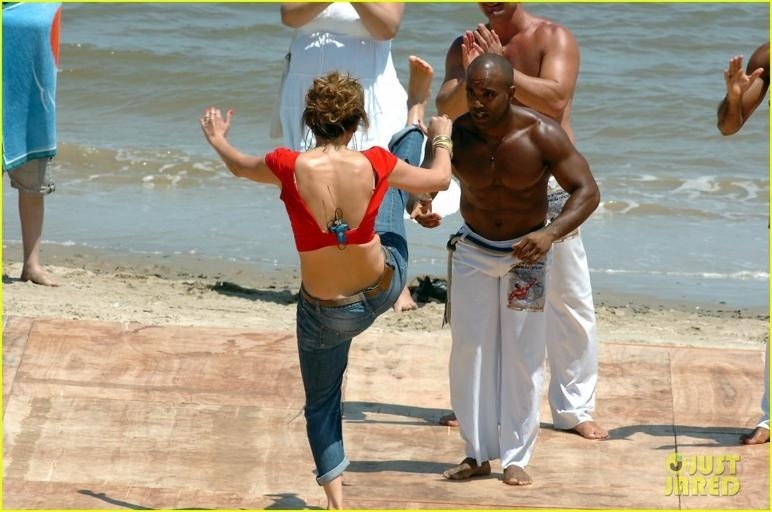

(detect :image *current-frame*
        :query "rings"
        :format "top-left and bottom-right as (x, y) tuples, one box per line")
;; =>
(210, 112), (216, 115)
(204, 115), (210, 118)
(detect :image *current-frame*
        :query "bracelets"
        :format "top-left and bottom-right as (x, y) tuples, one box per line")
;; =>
(433, 136), (453, 159)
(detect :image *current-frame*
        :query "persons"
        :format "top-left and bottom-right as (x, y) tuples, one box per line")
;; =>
(437, 2), (609, 437)
(280, 2), (418, 313)
(195, 55), (453, 510)
(508, 279), (535, 304)
(2, 2), (60, 287)
(717, 41), (770, 444)
(407, 53), (600, 485)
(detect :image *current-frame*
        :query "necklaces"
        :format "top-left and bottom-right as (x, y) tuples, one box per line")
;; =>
(488, 152), (498, 161)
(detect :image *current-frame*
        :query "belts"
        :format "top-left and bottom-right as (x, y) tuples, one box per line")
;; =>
(300, 264), (394, 307)
